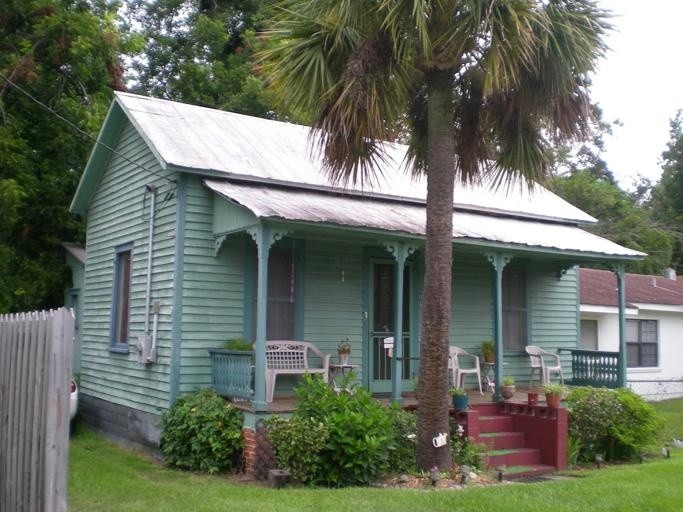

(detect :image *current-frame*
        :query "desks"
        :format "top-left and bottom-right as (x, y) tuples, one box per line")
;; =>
(329, 363), (359, 396)
(480, 363), (510, 395)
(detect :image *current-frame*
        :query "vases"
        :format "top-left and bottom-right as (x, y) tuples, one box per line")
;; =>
(527, 392), (538, 404)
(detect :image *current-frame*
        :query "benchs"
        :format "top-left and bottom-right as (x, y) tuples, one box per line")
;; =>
(250, 339), (329, 404)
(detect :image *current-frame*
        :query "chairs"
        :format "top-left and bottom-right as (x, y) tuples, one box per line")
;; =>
(524, 345), (563, 390)
(447, 345), (484, 396)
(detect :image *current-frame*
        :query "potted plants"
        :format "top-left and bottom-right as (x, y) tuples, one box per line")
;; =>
(500, 376), (516, 399)
(540, 382), (566, 407)
(448, 386), (469, 411)
(480, 340), (495, 363)
(336, 335), (351, 364)
